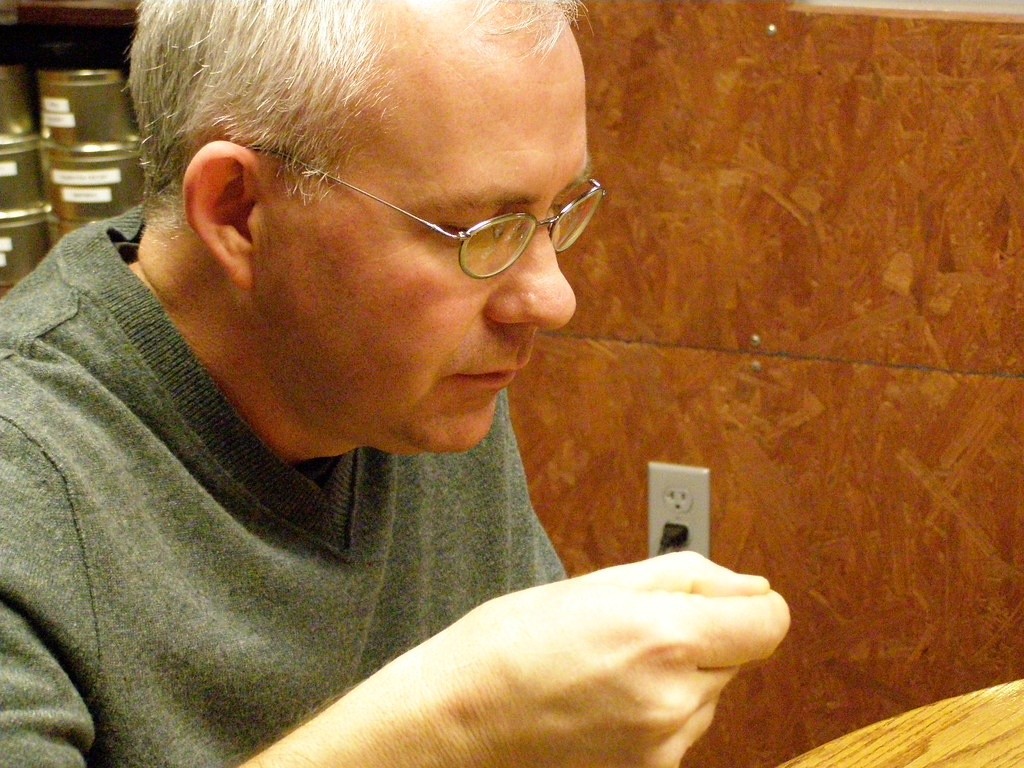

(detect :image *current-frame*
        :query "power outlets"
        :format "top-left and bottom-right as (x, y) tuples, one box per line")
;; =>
(648, 464), (712, 560)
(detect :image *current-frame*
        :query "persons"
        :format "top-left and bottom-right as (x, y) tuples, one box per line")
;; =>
(1, 0), (792, 768)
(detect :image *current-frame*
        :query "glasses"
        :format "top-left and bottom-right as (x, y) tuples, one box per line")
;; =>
(244, 142), (605, 280)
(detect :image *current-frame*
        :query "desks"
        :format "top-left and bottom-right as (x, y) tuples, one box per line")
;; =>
(770, 678), (1024, 767)
